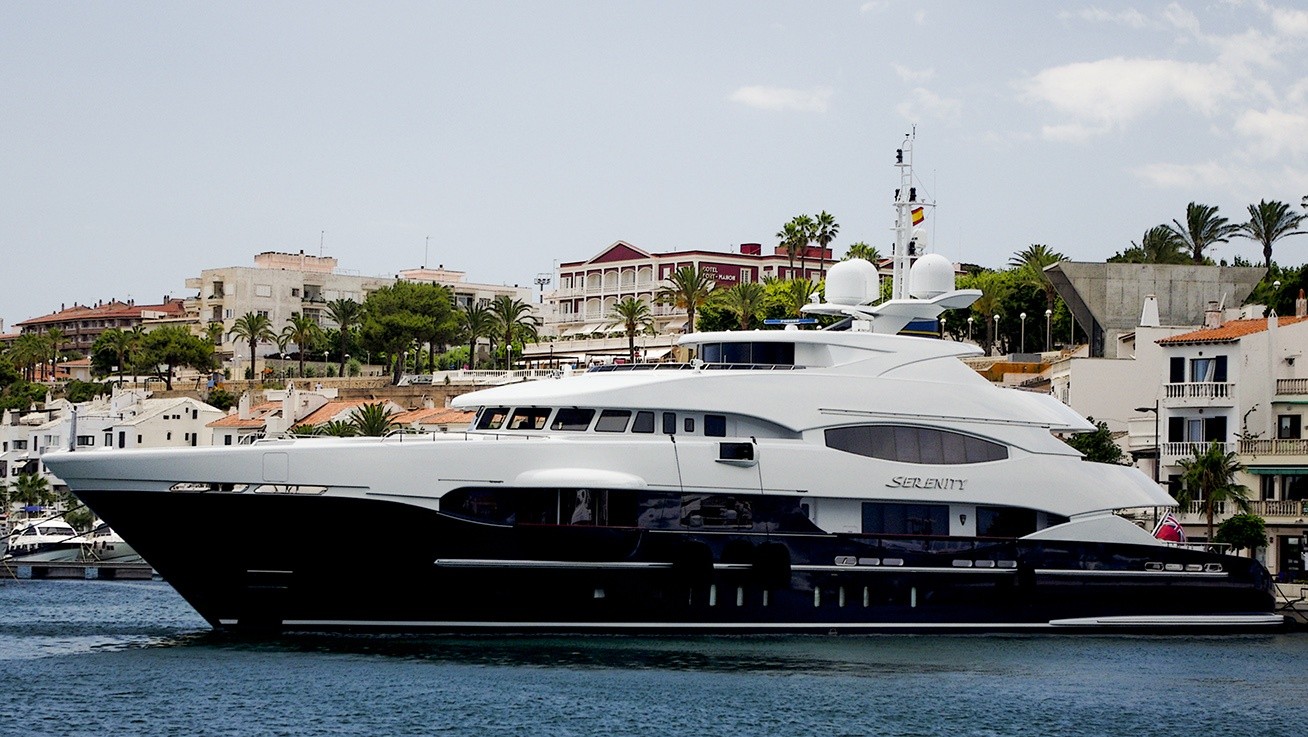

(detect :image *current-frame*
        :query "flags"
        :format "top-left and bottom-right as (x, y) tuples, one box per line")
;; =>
(1154, 514), (1187, 543)
(911, 206), (924, 225)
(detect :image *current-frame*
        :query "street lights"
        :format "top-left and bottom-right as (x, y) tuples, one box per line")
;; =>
(967, 317), (974, 340)
(87, 356), (92, 382)
(1135, 399), (1159, 485)
(324, 351), (330, 377)
(1045, 308), (1052, 352)
(506, 344), (512, 370)
(940, 318), (947, 340)
(280, 353), (285, 378)
(63, 356), (67, 382)
(993, 314), (1000, 345)
(641, 334), (647, 363)
(48, 358), (54, 372)
(1020, 312), (1026, 353)
(404, 351), (408, 374)
(230, 357), (235, 380)
(237, 354), (242, 380)
(669, 332), (675, 361)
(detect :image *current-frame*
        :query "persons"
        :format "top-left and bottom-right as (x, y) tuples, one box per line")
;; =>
(589, 360), (594, 367)
(464, 363), (469, 370)
(572, 362), (576, 369)
(449, 364), (456, 370)
(541, 362), (544, 368)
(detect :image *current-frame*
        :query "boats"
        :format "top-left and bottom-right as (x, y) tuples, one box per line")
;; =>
(0, 490), (143, 563)
(40, 120), (1286, 651)
